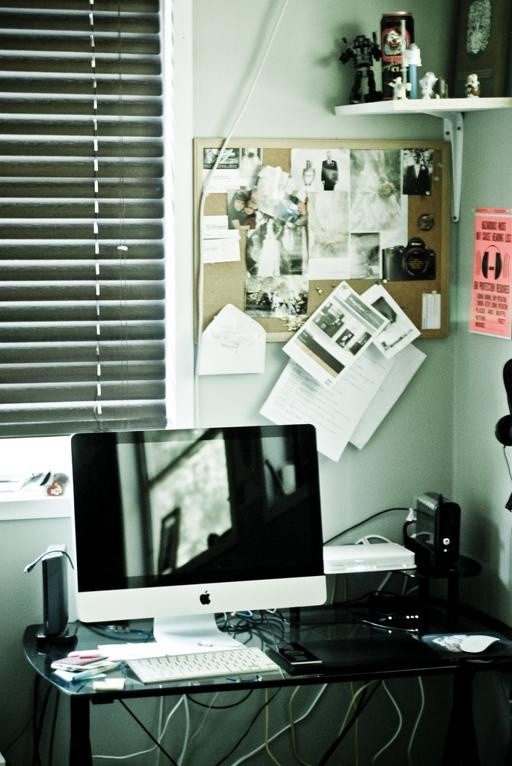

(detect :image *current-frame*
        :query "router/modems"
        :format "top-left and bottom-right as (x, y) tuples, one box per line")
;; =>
(323, 542), (415, 573)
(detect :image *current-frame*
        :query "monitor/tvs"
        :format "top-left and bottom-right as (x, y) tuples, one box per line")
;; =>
(72, 423), (327, 662)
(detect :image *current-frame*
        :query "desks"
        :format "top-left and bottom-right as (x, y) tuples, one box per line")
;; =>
(23, 592), (511, 765)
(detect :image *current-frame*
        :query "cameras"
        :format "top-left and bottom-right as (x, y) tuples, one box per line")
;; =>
(383, 236), (436, 281)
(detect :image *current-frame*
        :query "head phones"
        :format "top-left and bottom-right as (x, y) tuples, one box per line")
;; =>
(494, 358), (512, 447)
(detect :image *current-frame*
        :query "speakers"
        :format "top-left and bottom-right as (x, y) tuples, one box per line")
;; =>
(417, 491), (461, 564)
(42, 545), (69, 636)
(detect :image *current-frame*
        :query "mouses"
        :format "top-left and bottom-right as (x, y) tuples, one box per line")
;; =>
(460, 633), (500, 656)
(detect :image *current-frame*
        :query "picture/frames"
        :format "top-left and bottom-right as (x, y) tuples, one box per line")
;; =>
(192, 136), (453, 341)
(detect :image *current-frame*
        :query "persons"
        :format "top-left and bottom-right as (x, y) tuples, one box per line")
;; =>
(242, 200), (255, 228)
(228, 191), (250, 231)
(340, 31), (380, 104)
(322, 151), (338, 190)
(465, 74), (481, 97)
(403, 152), (431, 194)
(303, 161), (315, 185)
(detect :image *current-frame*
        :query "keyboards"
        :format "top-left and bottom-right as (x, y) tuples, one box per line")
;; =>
(127, 646), (282, 686)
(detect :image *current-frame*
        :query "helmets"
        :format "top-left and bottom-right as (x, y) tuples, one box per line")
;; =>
(232, 190), (249, 201)
